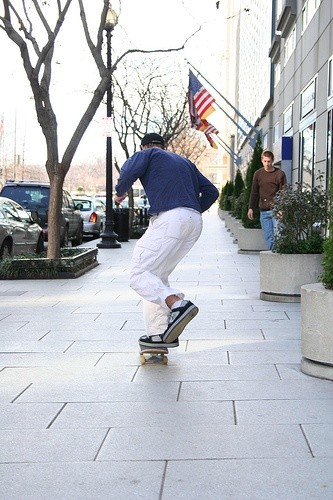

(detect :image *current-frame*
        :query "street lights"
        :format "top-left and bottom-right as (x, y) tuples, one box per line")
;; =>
(95, 8), (122, 249)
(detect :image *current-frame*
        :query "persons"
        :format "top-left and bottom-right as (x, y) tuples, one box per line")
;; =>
(247, 151), (287, 250)
(114, 133), (219, 349)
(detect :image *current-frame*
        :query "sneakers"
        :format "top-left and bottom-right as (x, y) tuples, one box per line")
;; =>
(163, 301), (199, 343)
(138, 334), (179, 348)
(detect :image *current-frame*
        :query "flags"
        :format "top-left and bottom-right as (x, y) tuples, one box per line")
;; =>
(188, 69), (219, 150)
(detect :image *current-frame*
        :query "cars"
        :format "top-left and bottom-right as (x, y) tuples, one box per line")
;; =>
(0, 197), (45, 259)
(72, 196), (150, 239)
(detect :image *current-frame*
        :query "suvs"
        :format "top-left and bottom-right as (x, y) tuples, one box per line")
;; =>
(0, 183), (84, 248)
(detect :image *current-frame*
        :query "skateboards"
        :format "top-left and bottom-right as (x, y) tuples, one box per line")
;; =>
(139, 344), (169, 366)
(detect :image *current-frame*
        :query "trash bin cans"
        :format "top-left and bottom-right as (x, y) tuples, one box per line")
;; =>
(112, 208), (130, 242)
